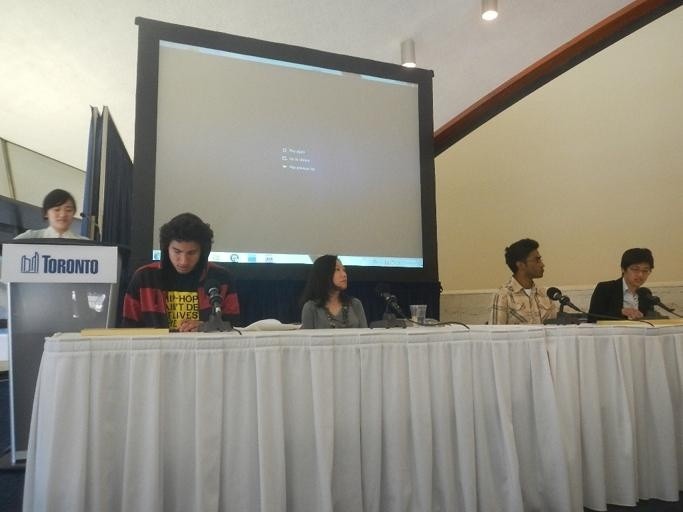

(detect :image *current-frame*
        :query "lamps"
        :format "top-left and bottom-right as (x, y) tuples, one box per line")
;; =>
(480, 1), (498, 21)
(400, 39), (417, 69)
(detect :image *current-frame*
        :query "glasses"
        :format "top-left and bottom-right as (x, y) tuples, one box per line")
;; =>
(628, 265), (651, 275)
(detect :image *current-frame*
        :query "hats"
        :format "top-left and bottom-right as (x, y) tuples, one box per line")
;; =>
(159, 212), (213, 282)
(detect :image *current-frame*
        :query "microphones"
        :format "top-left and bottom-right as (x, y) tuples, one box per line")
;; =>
(376, 285), (407, 318)
(80, 212), (100, 243)
(638, 287), (671, 311)
(204, 279), (223, 315)
(547, 288), (579, 311)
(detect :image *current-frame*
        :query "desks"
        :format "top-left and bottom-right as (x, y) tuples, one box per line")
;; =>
(21, 316), (683, 512)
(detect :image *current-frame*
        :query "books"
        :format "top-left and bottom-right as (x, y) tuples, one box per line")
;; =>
(595, 318), (682, 326)
(81, 327), (171, 337)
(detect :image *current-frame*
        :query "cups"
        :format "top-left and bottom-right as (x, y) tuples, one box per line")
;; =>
(409, 305), (427, 327)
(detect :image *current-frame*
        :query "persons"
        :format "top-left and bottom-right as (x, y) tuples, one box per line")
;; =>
(299, 254), (370, 330)
(486, 238), (559, 324)
(119, 212), (242, 332)
(13, 188), (91, 240)
(588, 248), (654, 320)
